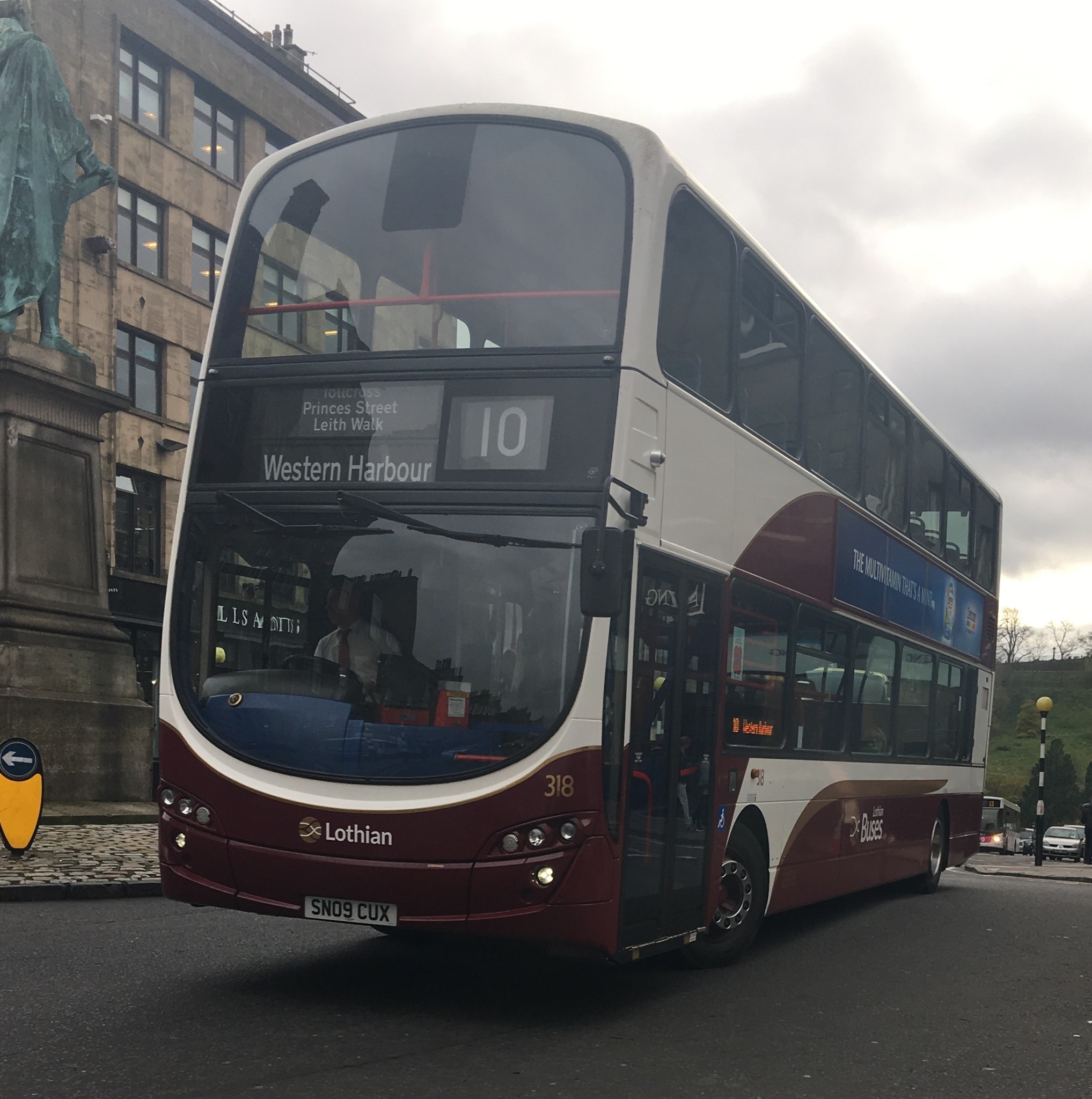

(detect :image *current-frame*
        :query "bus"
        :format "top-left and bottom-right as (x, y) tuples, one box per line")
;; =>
(979, 796), (1020, 856)
(160, 103), (1000, 972)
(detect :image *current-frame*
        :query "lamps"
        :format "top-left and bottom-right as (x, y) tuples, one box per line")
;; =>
(156, 438), (187, 453)
(84, 235), (117, 264)
(90, 114), (112, 126)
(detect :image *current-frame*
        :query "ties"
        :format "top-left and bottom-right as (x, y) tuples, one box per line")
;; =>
(337, 628), (351, 666)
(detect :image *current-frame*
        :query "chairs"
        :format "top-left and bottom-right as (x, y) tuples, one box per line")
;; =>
(668, 351), (992, 591)
(805, 723), (957, 760)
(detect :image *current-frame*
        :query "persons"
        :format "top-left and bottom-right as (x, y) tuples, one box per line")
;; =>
(725, 676), (803, 747)
(0, 0), (117, 361)
(310, 583), (402, 701)
(671, 735), (702, 833)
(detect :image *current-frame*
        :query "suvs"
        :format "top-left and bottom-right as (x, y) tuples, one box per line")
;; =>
(1063, 824), (1085, 859)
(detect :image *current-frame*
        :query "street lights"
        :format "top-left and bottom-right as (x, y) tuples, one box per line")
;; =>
(1034, 697), (1053, 866)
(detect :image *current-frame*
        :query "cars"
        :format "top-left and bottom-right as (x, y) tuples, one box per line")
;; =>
(1042, 826), (1084, 863)
(1019, 828), (1036, 856)
